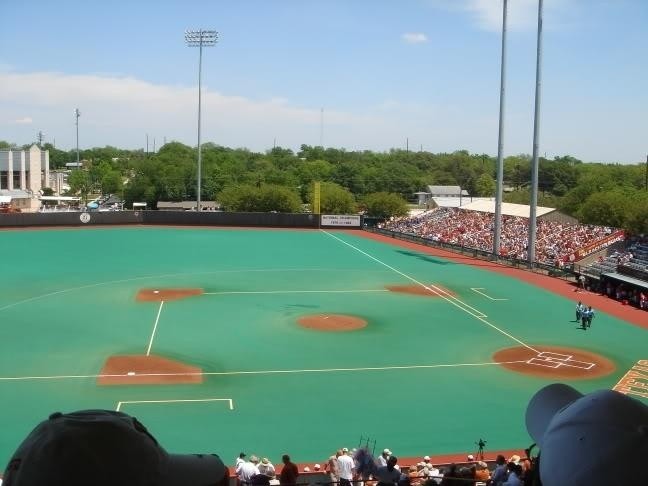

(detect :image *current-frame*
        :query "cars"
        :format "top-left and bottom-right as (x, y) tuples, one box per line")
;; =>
(0, 202), (22, 214)
(40, 169), (120, 212)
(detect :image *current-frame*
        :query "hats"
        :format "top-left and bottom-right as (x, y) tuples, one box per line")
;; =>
(477, 461), (488, 469)
(423, 455), (431, 461)
(303, 467), (311, 472)
(0, 392), (229, 486)
(239, 453), (247, 459)
(342, 447), (348, 452)
(416, 461), (427, 468)
(383, 448), (393, 457)
(314, 464), (321, 469)
(260, 457), (272, 468)
(508, 453), (521, 464)
(248, 455), (260, 463)
(425, 463), (433, 470)
(467, 454), (474, 460)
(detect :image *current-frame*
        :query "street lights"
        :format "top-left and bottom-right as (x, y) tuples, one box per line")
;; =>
(183, 27), (219, 212)
(73, 107), (81, 171)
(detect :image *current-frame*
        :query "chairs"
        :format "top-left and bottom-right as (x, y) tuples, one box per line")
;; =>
(584, 240), (647, 283)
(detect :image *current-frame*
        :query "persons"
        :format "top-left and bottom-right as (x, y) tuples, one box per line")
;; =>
(0, 411), (225, 486)
(376, 207), (648, 330)
(235, 447), (532, 486)
